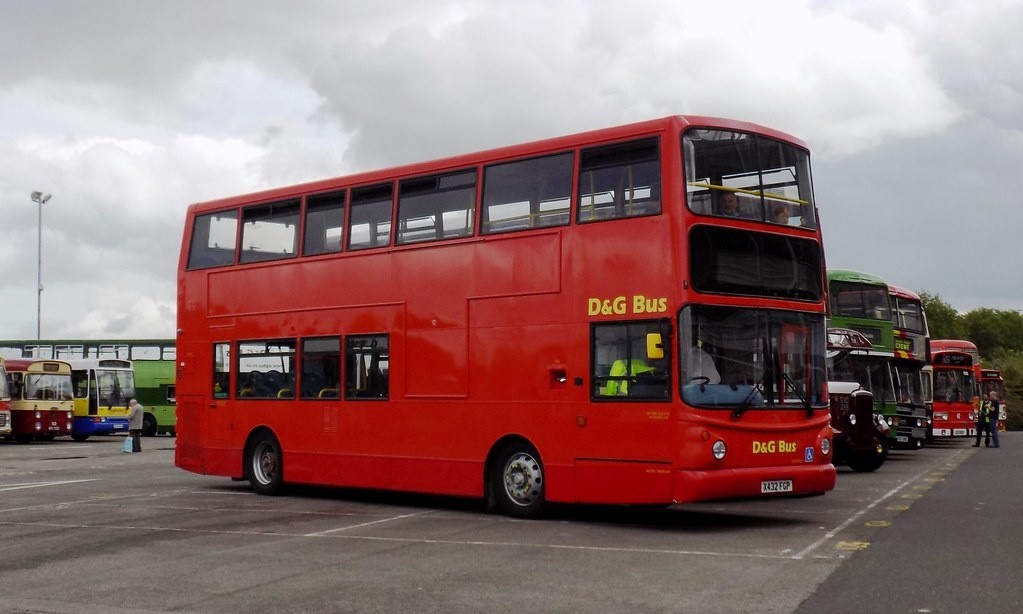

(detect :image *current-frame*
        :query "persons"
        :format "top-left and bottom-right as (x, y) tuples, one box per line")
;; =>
(370, 358), (379, 370)
(768, 201), (806, 227)
(713, 191), (742, 219)
(984, 390), (999, 448)
(124, 398), (144, 453)
(239, 372), (257, 396)
(692, 344), (722, 385)
(972, 393), (992, 447)
(644, 197), (659, 213)
(852, 300), (877, 318)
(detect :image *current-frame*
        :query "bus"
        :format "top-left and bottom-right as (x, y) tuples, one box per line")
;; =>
(4, 360), (74, 442)
(929, 338), (982, 438)
(61, 358), (136, 441)
(824, 270), (900, 441)
(887, 286), (935, 448)
(753, 326), (891, 473)
(0, 339), (177, 436)
(0, 359), (13, 436)
(173, 114), (836, 518)
(981, 368), (1007, 433)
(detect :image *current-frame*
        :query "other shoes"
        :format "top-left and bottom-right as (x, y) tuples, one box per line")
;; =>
(985, 443), (990, 447)
(972, 444), (980, 447)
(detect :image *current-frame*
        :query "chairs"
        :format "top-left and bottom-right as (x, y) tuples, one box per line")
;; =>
(239, 370), (366, 398)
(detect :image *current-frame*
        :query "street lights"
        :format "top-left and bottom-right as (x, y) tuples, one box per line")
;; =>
(30, 191), (52, 339)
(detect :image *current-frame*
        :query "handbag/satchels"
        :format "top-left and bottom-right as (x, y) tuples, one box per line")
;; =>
(122, 437), (133, 453)
